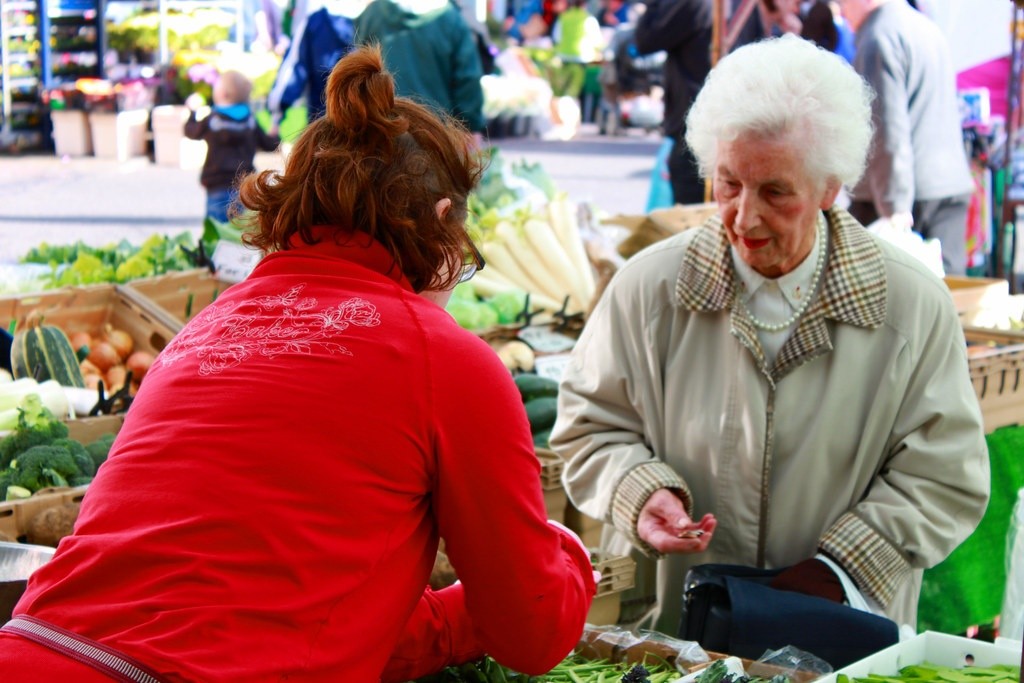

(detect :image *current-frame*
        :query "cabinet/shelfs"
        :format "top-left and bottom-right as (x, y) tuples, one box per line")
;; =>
(0, 3), (249, 152)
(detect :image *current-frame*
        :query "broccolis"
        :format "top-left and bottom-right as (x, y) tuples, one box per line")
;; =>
(0, 393), (116, 501)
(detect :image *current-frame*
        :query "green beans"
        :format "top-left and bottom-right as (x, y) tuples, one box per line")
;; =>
(544, 652), (679, 683)
(837, 664), (1020, 683)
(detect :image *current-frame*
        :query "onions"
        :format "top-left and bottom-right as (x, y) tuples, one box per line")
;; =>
(68, 323), (153, 403)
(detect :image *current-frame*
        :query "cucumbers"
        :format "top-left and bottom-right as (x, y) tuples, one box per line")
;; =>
(514, 376), (559, 447)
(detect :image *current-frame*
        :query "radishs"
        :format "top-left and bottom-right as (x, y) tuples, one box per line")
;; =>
(469, 201), (597, 313)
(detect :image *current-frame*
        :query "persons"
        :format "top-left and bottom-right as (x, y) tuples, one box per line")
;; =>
(0, 0), (612, 683)
(549, 32), (990, 638)
(599, 0), (977, 281)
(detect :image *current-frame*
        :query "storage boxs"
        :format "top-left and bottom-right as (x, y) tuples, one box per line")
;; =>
(0, 201), (1024, 683)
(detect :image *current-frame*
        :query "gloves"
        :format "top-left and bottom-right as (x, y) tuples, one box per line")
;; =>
(764, 549), (860, 605)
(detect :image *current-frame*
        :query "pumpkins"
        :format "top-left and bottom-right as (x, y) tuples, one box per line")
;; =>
(10, 326), (84, 389)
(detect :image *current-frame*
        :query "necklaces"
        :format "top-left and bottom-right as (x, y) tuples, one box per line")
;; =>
(737, 215), (826, 332)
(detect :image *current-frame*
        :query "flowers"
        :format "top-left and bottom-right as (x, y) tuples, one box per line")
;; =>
(482, 74), (554, 123)
(2, 6), (224, 146)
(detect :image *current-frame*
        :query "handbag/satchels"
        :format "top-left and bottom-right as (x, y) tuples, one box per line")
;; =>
(678, 562), (900, 673)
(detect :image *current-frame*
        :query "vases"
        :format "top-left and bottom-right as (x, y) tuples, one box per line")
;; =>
(484, 119), (538, 137)
(48, 105), (207, 166)
